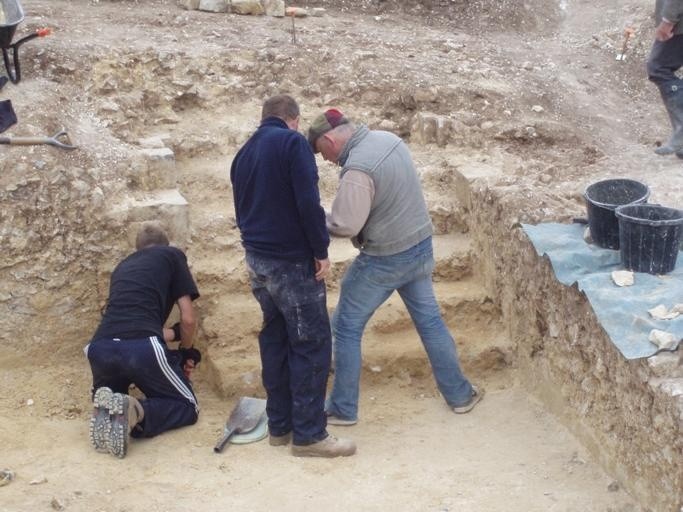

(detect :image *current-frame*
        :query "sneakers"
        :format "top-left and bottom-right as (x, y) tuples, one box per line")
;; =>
(109, 392), (145, 459)
(454, 386), (481, 413)
(90, 387), (113, 453)
(325, 410), (356, 426)
(270, 433), (356, 457)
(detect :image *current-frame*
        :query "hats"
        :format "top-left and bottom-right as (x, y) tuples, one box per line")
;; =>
(309, 109), (348, 153)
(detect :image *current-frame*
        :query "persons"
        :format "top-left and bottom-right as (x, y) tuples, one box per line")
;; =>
(308, 107), (487, 429)
(85, 223), (201, 459)
(228, 93), (358, 459)
(643, 1), (683, 156)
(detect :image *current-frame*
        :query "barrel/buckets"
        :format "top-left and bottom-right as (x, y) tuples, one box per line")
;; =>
(615, 203), (683, 275)
(582, 178), (650, 249)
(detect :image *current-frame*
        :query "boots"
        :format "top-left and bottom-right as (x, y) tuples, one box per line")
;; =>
(653, 79), (682, 155)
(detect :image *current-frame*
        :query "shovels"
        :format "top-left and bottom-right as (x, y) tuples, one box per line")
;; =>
(214, 397), (267, 452)
